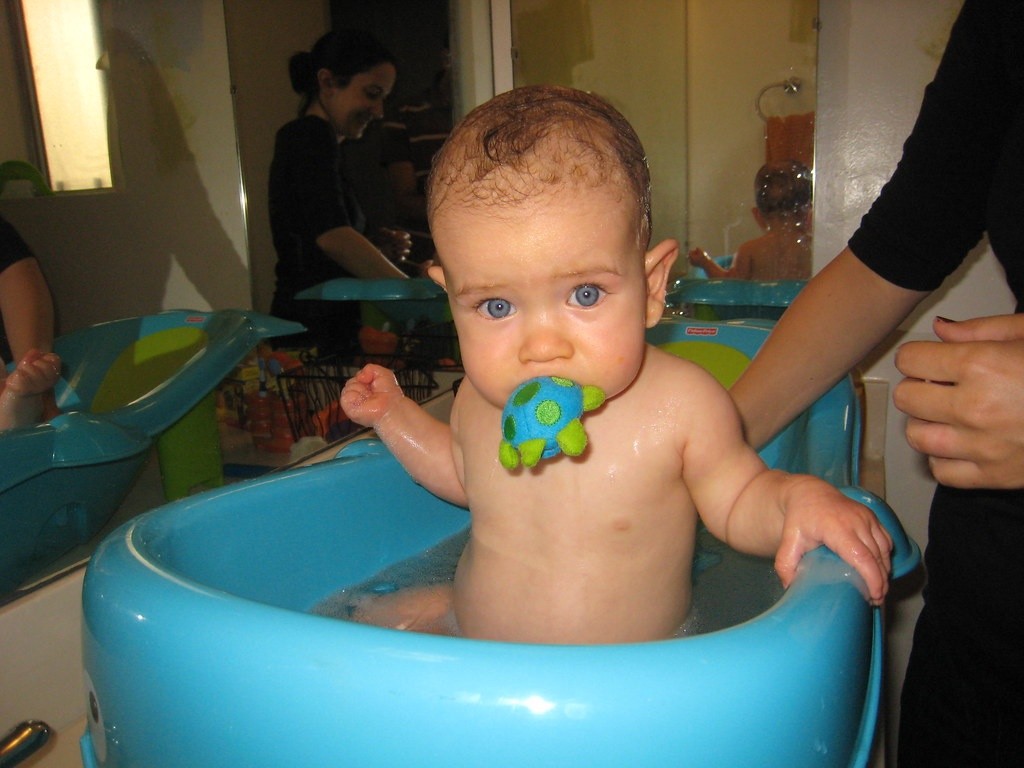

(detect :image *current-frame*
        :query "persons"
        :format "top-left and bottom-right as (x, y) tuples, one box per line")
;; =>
(339, 83), (894, 646)
(268, 21), (457, 356)
(0, 213), (73, 431)
(725, 0), (1024, 696)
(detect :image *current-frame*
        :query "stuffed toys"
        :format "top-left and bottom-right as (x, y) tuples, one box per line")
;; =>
(497, 374), (606, 470)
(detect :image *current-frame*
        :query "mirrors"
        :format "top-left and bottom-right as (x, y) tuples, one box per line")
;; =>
(0, 1), (820, 608)
